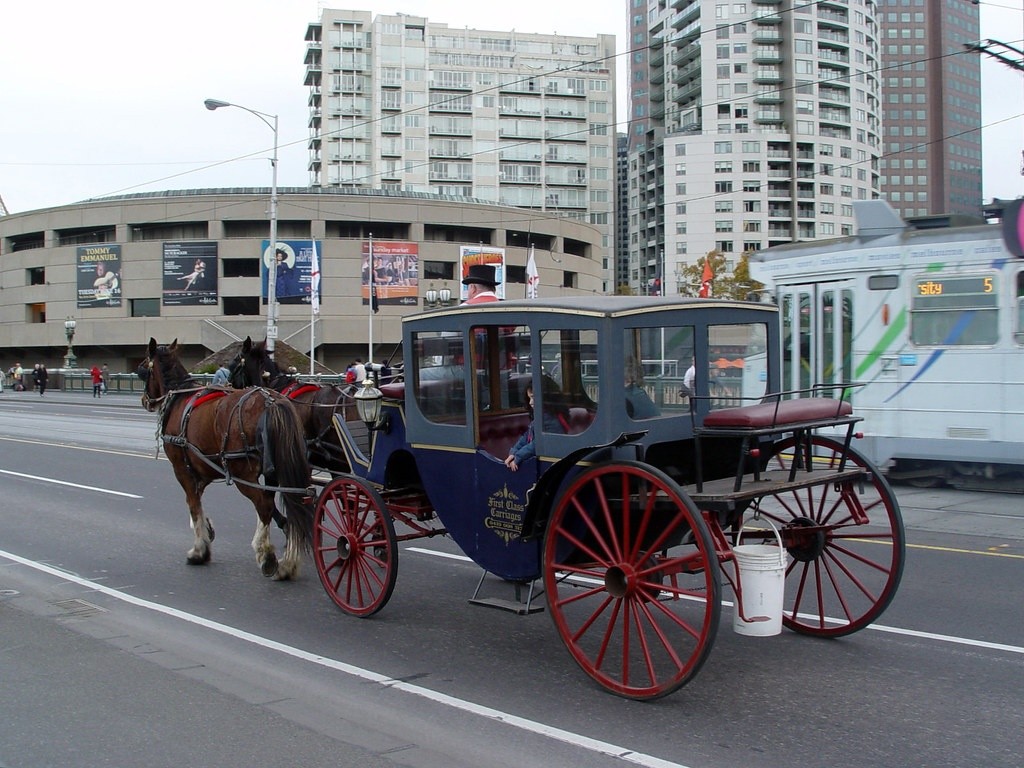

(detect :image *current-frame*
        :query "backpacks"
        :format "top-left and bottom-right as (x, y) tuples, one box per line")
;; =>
(346, 371), (356, 383)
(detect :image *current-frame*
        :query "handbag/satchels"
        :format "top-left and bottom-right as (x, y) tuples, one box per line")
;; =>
(100, 381), (106, 391)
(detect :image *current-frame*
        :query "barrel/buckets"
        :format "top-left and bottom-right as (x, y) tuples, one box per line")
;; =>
(731, 516), (787, 637)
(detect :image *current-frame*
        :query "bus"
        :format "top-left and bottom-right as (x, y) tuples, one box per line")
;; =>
(737, 198), (1024, 482)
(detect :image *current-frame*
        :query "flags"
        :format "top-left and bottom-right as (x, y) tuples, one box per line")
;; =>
(526, 256), (540, 299)
(313, 241), (321, 316)
(372, 257), (379, 314)
(699, 260), (714, 298)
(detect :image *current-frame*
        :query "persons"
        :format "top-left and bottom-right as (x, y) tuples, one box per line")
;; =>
(177, 258), (206, 292)
(101, 363), (109, 395)
(345, 359), (368, 387)
(90, 367), (103, 398)
(13, 362), (23, 384)
(568, 352), (662, 421)
(0, 368), (6, 393)
(31, 363), (50, 396)
(434, 264), (517, 413)
(550, 353), (561, 376)
(380, 359), (392, 385)
(364, 254), (399, 286)
(212, 362), (232, 385)
(683, 354), (696, 390)
(93, 261), (119, 299)
(503, 376), (572, 471)
(275, 248), (288, 266)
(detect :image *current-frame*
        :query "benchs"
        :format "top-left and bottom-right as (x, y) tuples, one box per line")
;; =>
(688, 381), (869, 493)
(445, 408), (592, 458)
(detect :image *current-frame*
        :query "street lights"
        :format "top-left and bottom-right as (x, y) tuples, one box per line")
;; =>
(62, 315), (77, 369)
(201, 97), (281, 363)
(426, 281), (451, 337)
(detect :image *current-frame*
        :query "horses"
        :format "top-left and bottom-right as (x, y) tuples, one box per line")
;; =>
(141, 336), (318, 581)
(224, 334), (387, 571)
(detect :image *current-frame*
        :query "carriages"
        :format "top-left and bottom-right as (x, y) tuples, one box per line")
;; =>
(136, 292), (910, 708)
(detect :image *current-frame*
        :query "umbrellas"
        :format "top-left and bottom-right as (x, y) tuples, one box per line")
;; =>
(713, 357), (745, 377)
